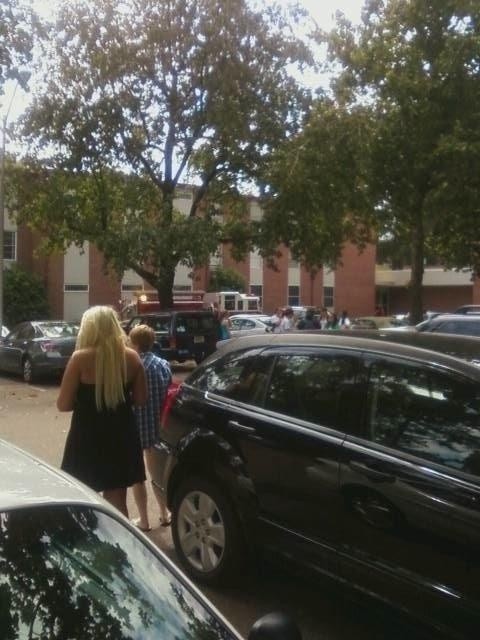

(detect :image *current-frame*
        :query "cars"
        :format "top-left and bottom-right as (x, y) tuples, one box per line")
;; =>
(0, 319), (82, 386)
(0, 435), (302, 640)
(218, 314), (283, 338)
(275, 306), (340, 329)
(456, 304), (480, 313)
(406, 313), (478, 342)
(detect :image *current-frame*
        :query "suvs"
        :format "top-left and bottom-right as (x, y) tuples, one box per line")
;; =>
(148, 328), (479, 640)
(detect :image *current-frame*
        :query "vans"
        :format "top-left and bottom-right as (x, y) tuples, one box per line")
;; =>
(122, 307), (222, 366)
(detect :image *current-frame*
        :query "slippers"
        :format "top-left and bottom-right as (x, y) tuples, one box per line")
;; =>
(158, 512), (174, 528)
(131, 517), (153, 533)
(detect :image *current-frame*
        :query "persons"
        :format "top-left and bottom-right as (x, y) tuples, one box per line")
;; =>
(129, 325), (171, 531)
(211, 302), (232, 340)
(272, 308), (350, 333)
(57, 306), (146, 518)
